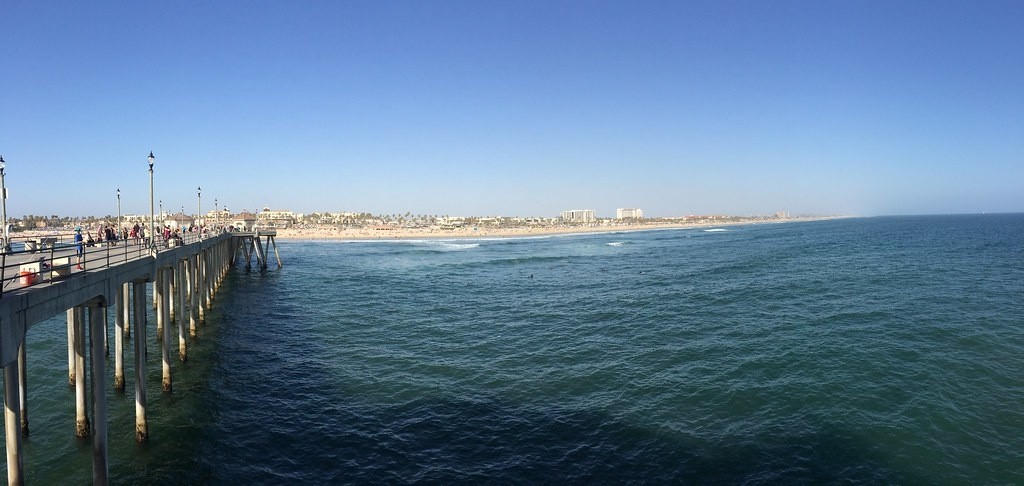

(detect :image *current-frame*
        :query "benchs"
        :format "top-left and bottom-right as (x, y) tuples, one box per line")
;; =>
(83, 243), (101, 247)
(20, 257), (71, 282)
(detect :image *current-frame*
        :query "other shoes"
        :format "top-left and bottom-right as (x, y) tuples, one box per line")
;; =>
(76, 267), (83, 271)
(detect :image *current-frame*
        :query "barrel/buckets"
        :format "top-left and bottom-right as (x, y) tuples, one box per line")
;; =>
(25, 241), (36, 251)
(168, 238), (176, 248)
(19, 272), (32, 286)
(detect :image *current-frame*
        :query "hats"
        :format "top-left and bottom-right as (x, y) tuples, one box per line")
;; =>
(74, 228), (80, 231)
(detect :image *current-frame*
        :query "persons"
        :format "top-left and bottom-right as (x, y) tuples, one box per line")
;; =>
(74, 226), (84, 270)
(85, 218), (246, 249)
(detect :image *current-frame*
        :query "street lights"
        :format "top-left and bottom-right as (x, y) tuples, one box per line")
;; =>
(214, 198), (218, 230)
(196, 185), (202, 240)
(181, 206), (184, 233)
(0, 155), (14, 255)
(147, 150), (157, 252)
(158, 199), (163, 235)
(116, 187), (122, 240)
